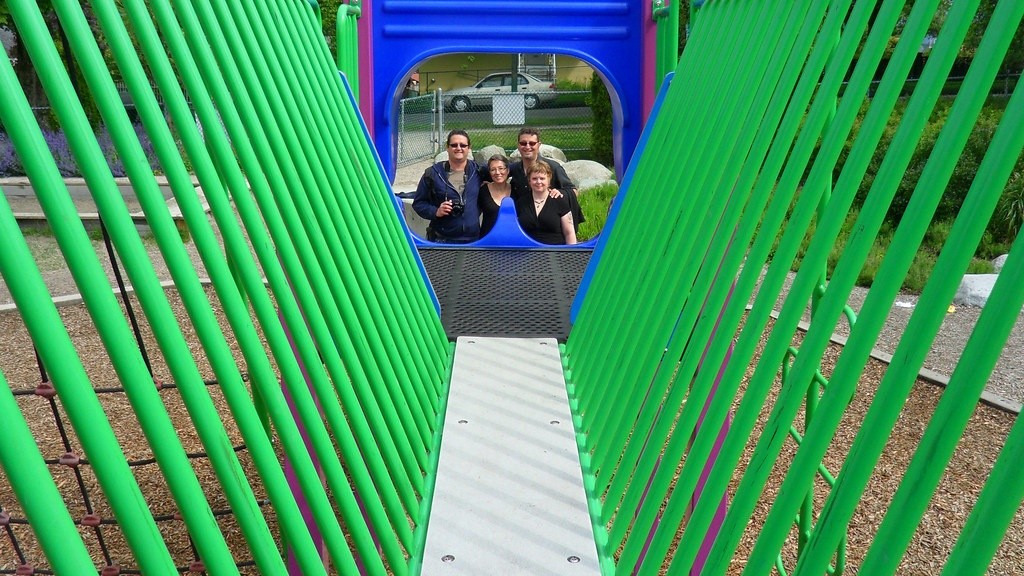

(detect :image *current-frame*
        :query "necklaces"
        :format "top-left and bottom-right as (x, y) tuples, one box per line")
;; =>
(534, 200), (545, 208)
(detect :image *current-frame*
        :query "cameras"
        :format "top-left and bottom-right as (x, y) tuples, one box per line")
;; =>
(451, 203), (465, 217)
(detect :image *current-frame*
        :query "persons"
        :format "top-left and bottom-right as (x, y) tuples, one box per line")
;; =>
(411, 128), (585, 245)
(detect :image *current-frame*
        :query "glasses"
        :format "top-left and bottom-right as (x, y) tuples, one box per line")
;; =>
(518, 141), (538, 146)
(449, 144), (469, 148)
(490, 167), (507, 172)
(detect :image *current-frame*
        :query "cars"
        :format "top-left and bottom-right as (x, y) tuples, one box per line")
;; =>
(444, 71), (557, 113)
